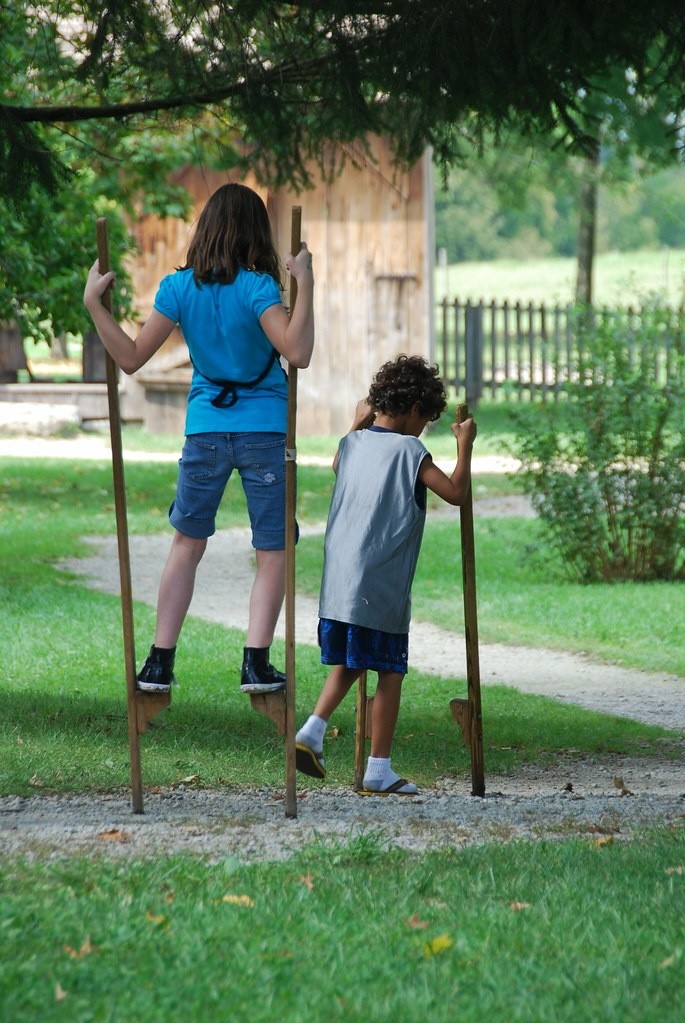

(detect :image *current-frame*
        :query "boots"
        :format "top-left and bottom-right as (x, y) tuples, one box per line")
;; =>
(236, 647), (287, 693)
(137, 643), (177, 693)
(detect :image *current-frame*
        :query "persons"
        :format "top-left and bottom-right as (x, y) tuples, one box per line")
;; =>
(295, 355), (477, 795)
(82, 183), (314, 696)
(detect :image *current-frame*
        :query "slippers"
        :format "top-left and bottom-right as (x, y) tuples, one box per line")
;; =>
(357, 778), (418, 798)
(294, 744), (326, 779)
